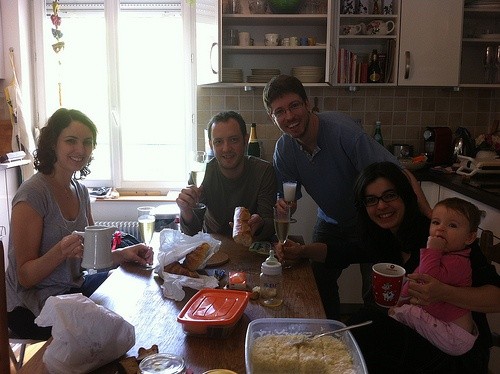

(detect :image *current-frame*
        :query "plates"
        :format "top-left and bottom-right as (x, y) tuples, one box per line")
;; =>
(465, 0), (500, 41)
(222, 66), (326, 84)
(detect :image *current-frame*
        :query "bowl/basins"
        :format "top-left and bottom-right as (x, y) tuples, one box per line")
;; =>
(268, 0), (305, 13)
(177, 288), (251, 338)
(245, 317), (368, 374)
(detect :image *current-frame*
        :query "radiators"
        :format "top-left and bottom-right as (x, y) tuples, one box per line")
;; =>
(95, 221), (142, 239)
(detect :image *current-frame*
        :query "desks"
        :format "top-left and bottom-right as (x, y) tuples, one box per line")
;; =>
(16, 233), (329, 374)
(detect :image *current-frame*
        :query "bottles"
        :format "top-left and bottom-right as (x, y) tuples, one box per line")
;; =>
(247, 123), (260, 158)
(367, 48), (382, 83)
(259, 250), (285, 306)
(375, 122), (384, 146)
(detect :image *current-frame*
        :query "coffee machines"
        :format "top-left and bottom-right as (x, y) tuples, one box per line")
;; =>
(423, 126), (453, 165)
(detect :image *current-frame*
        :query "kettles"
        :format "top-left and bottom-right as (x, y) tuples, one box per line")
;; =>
(453, 127), (476, 164)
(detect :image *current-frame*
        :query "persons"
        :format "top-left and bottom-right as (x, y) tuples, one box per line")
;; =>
(176, 112), (280, 240)
(387, 197), (481, 357)
(260, 75), (434, 328)
(275, 161), (500, 374)
(5, 108), (154, 342)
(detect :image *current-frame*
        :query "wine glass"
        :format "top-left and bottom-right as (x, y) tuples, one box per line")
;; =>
(282, 179), (298, 223)
(137, 205), (156, 270)
(482, 46), (500, 85)
(193, 151), (207, 209)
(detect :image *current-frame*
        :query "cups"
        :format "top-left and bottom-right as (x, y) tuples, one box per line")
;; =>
(393, 144), (413, 159)
(248, 0), (267, 13)
(239, 32), (315, 46)
(371, 263), (417, 309)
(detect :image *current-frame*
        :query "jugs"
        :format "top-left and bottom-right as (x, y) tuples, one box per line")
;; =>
(71, 224), (118, 269)
(341, 20), (395, 35)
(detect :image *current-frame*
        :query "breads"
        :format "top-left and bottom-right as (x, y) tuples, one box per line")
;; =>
(164, 243), (213, 277)
(232, 207), (253, 248)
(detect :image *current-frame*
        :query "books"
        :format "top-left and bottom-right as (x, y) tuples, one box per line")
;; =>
(338, 48), (367, 83)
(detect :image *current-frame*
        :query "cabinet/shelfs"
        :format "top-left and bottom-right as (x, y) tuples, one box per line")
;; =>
(217, 0), (500, 91)
(0, 168), (22, 271)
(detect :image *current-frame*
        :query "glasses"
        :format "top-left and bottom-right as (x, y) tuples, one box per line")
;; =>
(359, 189), (399, 207)
(270, 98), (306, 120)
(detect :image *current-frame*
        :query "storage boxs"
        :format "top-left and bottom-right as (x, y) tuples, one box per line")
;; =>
(244, 317), (367, 374)
(177, 288), (250, 339)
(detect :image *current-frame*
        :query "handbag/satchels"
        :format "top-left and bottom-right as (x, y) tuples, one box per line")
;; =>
(33, 292), (134, 374)
